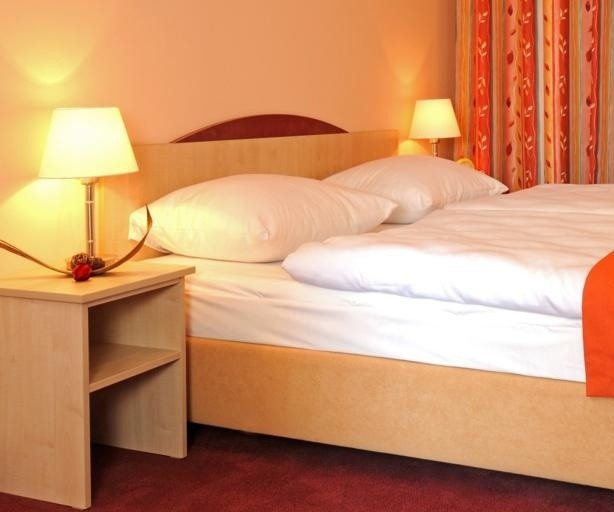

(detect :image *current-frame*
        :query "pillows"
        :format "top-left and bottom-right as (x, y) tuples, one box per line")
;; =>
(321, 154), (509, 226)
(128, 172), (398, 262)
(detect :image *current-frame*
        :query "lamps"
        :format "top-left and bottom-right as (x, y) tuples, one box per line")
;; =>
(38, 106), (139, 275)
(408, 97), (462, 158)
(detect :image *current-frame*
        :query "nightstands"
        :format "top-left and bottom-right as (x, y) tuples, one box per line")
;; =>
(1, 264), (196, 510)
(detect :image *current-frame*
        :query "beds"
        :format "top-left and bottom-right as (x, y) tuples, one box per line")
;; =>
(94, 129), (614, 492)
